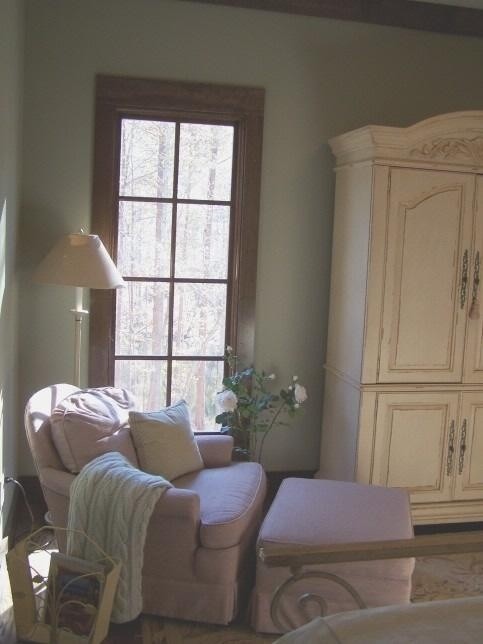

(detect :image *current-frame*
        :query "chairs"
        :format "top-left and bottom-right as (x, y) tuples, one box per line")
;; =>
(23, 383), (269, 626)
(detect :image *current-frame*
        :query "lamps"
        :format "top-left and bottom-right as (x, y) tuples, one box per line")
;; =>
(29, 228), (127, 388)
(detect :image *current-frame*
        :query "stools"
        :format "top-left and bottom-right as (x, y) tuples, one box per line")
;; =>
(251, 478), (416, 638)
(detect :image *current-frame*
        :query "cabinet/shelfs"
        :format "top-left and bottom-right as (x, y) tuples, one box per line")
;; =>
(328, 108), (483, 528)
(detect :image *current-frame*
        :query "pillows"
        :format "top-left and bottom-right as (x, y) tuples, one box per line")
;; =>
(51, 387), (204, 481)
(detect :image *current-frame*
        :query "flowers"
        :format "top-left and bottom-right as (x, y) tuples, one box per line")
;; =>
(215, 343), (308, 463)
